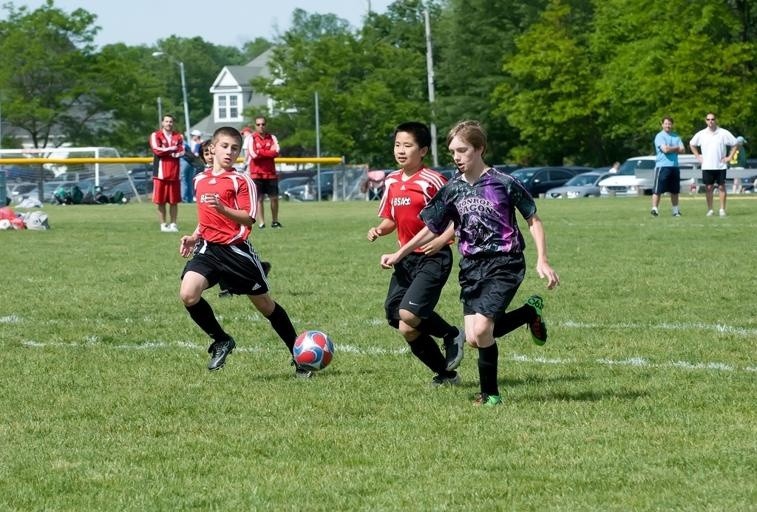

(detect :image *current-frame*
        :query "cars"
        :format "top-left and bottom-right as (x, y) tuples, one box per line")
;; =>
(490, 154), (757, 199)
(0, 163), (153, 205)
(264, 165), (461, 202)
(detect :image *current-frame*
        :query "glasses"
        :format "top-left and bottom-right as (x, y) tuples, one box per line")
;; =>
(256, 123), (266, 126)
(706, 119), (715, 121)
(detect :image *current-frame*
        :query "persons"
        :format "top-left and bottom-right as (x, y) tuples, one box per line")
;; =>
(248, 115), (280, 229)
(190, 129), (205, 175)
(179, 126), (315, 380)
(689, 111), (739, 217)
(199, 138), (214, 165)
(609, 162), (620, 174)
(367, 122), (466, 389)
(304, 162), (316, 200)
(179, 132), (194, 203)
(730, 135), (747, 194)
(380, 121), (559, 406)
(650, 117), (686, 217)
(239, 127), (252, 155)
(148, 114), (186, 232)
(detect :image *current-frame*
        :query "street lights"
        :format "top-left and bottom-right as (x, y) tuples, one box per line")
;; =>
(151, 52), (191, 147)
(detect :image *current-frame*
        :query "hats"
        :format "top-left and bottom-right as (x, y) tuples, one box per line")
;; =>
(190, 131), (201, 136)
(240, 128), (252, 135)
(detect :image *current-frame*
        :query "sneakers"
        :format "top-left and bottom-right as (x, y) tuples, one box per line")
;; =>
(441, 326), (465, 371)
(719, 209), (725, 216)
(259, 223), (265, 229)
(673, 208), (680, 216)
(293, 360), (312, 378)
(706, 209), (713, 216)
(271, 222), (282, 228)
(170, 224), (178, 232)
(161, 224), (172, 232)
(472, 393), (503, 406)
(525, 295), (546, 345)
(433, 371), (461, 386)
(651, 207), (658, 216)
(208, 337), (235, 370)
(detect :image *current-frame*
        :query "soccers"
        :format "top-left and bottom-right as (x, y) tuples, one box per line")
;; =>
(293, 331), (333, 371)
